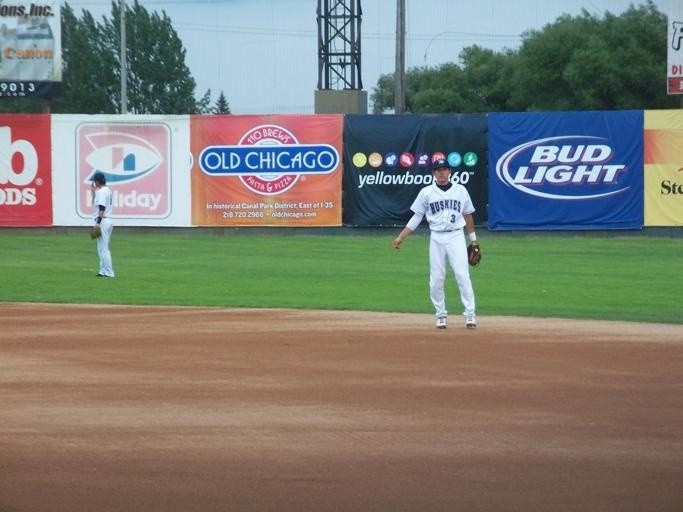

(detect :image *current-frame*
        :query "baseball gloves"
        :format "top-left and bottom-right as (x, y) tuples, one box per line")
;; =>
(467, 243), (481, 265)
(90, 226), (101, 239)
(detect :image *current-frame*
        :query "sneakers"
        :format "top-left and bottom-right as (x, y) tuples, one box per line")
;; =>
(436, 316), (447, 328)
(465, 315), (477, 327)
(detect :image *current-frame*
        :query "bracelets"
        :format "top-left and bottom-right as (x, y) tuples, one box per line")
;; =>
(94, 216), (102, 225)
(469, 231), (478, 243)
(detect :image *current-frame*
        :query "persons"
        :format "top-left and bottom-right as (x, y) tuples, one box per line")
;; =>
(390, 159), (481, 332)
(87, 170), (115, 279)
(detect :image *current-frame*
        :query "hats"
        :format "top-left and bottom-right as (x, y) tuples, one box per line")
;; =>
(432, 159), (450, 169)
(88, 170), (104, 180)
